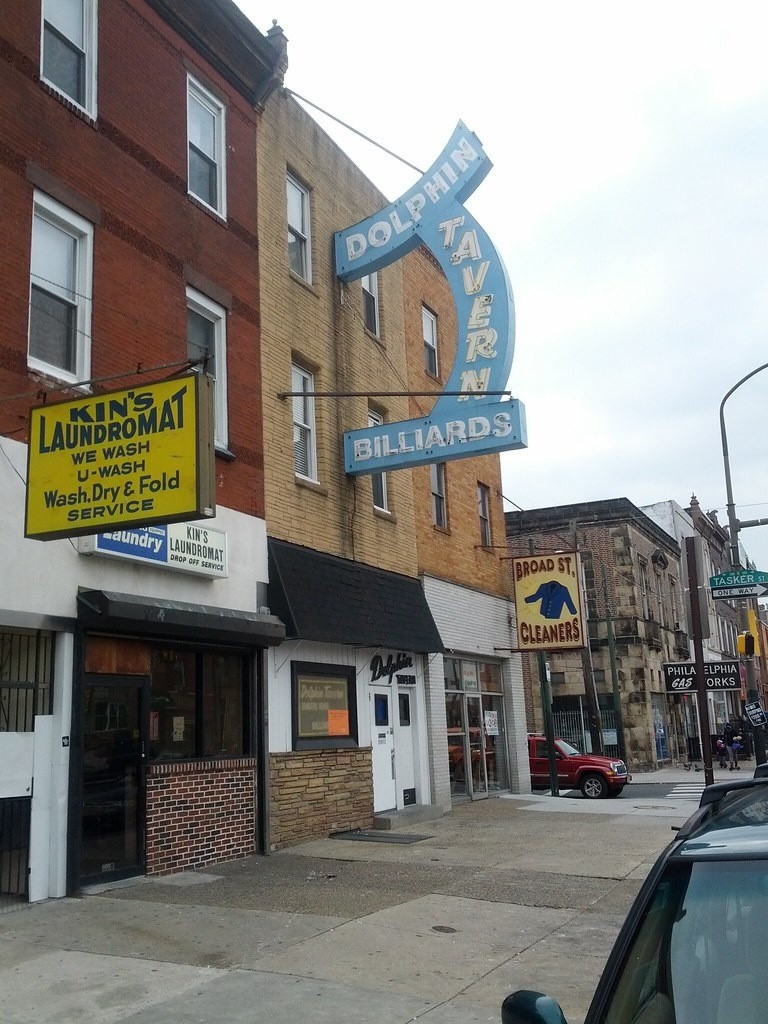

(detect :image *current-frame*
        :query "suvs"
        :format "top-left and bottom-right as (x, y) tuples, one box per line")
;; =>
(526, 734), (632, 799)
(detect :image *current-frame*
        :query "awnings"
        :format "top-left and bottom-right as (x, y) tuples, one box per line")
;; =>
(267, 538), (446, 655)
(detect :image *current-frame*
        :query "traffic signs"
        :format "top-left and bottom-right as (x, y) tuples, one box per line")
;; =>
(711, 582), (768, 600)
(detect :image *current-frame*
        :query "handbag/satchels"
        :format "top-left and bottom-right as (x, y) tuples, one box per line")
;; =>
(731, 740), (741, 751)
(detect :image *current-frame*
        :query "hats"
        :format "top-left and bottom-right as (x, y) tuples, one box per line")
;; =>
(726, 723), (731, 729)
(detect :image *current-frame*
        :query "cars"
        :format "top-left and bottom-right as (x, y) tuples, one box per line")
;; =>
(446, 726), (497, 779)
(503, 774), (768, 1024)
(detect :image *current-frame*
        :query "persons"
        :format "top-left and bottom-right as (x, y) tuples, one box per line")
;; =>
(716, 723), (740, 771)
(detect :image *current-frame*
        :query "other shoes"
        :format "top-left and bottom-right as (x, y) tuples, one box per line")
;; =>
(729, 762), (733, 771)
(724, 764), (727, 768)
(719, 764), (724, 768)
(733, 761), (740, 770)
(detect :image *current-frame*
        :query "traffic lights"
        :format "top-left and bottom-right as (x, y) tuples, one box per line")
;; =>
(737, 635), (754, 655)
